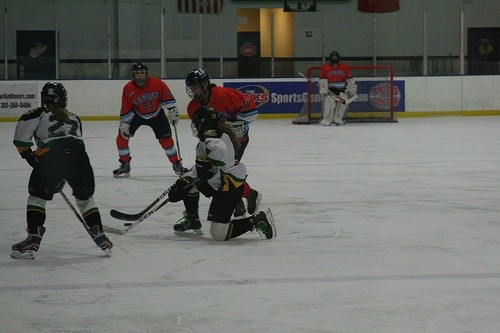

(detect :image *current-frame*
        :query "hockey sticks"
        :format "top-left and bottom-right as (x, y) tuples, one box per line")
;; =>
(172, 120), (184, 175)
(110, 165), (197, 221)
(298, 72), (357, 105)
(59, 190), (90, 232)
(101, 167), (213, 235)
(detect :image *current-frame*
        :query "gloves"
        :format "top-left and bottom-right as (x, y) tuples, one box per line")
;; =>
(225, 120), (244, 141)
(169, 179), (194, 203)
(119, 122), (131, 140)
(167, 108), (179, 126)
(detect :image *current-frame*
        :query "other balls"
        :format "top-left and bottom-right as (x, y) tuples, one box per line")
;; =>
(125, 223), (132, 226)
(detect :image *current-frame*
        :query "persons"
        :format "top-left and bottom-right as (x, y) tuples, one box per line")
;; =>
(10, 80), (114, 260)
(112, 62), (187, 178)
(186, 68), (262, 218)
(168, 106), (277, 242)
(318, 51), (357, 126)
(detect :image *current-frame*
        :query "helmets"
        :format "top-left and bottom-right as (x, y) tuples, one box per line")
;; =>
(330, 51), (340, 63)
(41, 82), (68, 107)
(185, 68), (209, 87)
(192, 106), (219, 125)
(131, 62), (149, 73)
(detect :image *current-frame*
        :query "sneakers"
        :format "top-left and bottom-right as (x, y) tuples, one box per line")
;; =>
(244, 188), (262, 216)
(251, 207), (277, 240)
(172, 160), (189, 175)
(10, 225), (46, 259)
(233, 198), (246, 220)
(87, 225), (113, 256)
(113, 157), (131, 178)
(174, 206), (204, 237)
